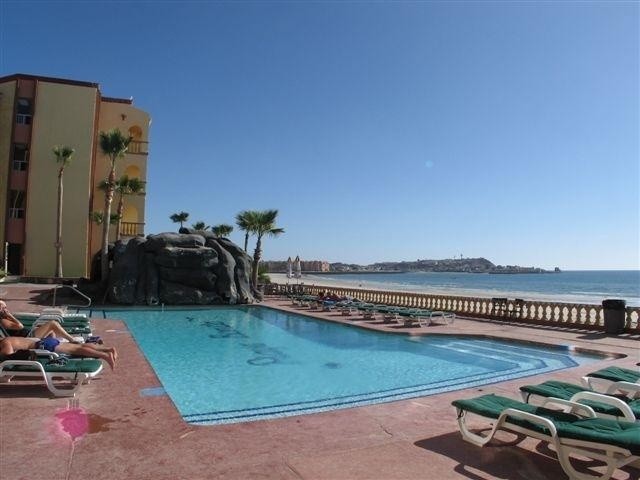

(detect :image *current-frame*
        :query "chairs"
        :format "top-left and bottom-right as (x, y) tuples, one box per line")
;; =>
(288, 295), (456, 328)
(0, 312), (103, 397)
(451, 365), (639, 480)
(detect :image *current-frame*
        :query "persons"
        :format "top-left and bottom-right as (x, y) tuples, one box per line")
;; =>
(314, 289), (365, 303)
(0, 300), (85, 344)
(0, 334), (118, 373)
